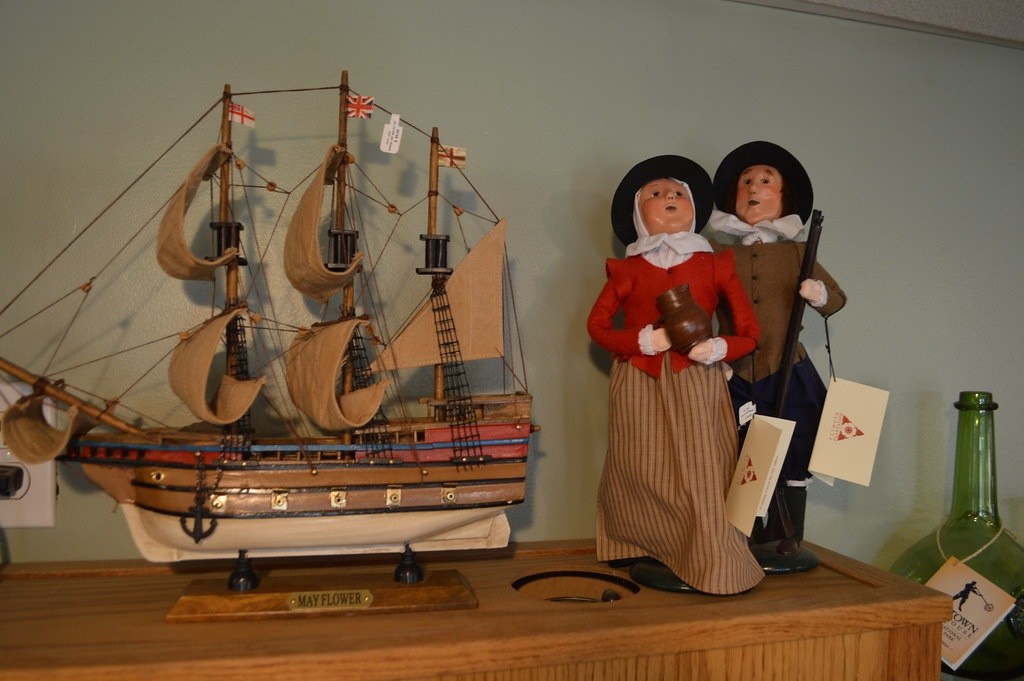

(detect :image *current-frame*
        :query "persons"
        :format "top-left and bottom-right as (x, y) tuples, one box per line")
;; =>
(587, 153), (764, 596)
(703, 140), (847, 558)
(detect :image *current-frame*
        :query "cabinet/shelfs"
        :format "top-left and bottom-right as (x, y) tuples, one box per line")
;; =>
(0, 537), (956, 681)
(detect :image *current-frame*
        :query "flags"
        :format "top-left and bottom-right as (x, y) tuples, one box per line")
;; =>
(347, 96), (373, 119)
(227, 102), (255, 126)
(438, 146), (466, 168)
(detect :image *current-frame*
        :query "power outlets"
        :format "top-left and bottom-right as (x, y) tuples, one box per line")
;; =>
(1, 384), (55, 529)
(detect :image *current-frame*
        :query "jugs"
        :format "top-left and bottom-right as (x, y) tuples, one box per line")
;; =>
(650, 282), (714, 354)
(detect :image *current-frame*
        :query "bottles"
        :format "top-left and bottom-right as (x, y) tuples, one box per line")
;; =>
(888, 391), (1024, 681)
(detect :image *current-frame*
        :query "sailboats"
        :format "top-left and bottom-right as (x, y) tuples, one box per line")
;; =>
(0, 71), (548, 591)
